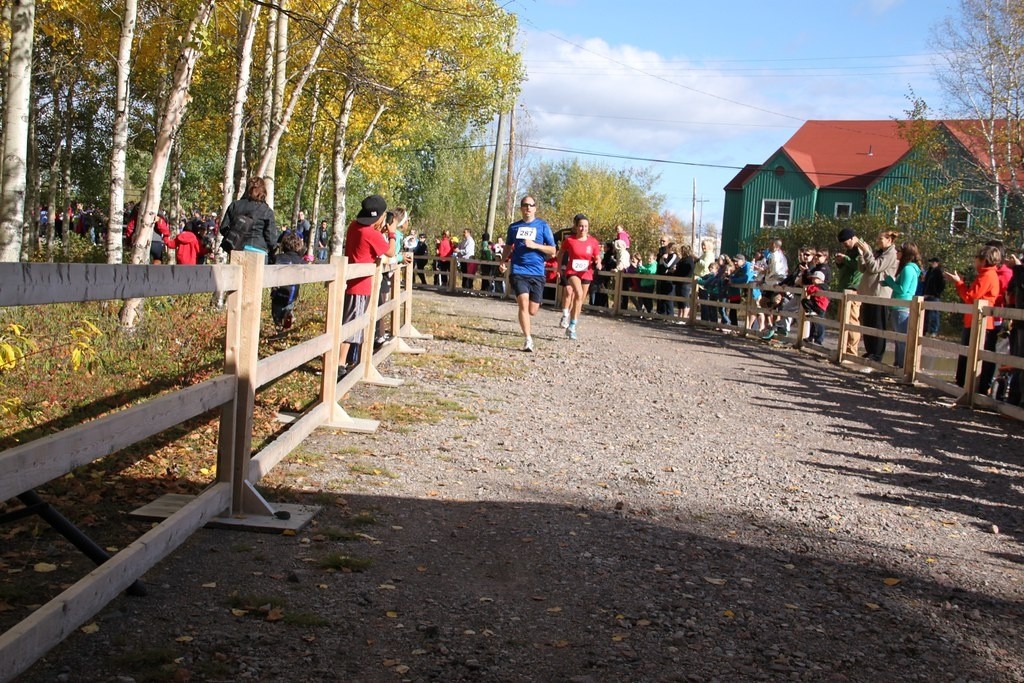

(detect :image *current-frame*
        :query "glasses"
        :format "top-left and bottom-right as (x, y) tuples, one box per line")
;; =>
(521, 203), (536, 208)
(803, 253), (813, 256)
(661, 240), (666, 242)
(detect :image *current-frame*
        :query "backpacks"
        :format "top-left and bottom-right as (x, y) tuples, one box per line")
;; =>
(221, 201), (267, 254)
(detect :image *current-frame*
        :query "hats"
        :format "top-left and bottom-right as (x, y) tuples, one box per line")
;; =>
(838, 228), (859, 242)
(732, 254), (745, 262)
(357, 197), (388, 225)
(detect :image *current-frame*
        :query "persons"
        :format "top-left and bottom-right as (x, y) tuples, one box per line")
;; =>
(497, 196), (558, 353)
(946, 243), (1024, 421)
(335, 195), (397, 379)
(855, 229), (901, 362)
(554, 214), (602, 338)
(694, 238), (828, 347)
(914, 256), (945, 337)
(548, 214), (587, 327)
(410, 228), (562, 311)
(828, 228), (872, 363)
(38, 177), (219, 272)
(371, 208), (411, 347)
(218, 177), (279, 267)
(877, 241), (922, 377)
(277, 211), (330, 260)
(264, 231), (309, 334)
(588, 224), (694, 325)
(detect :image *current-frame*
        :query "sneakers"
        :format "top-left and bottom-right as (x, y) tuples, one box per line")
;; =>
(566, 324), (577, 340)
(561, 309), (570, 328)
(522, 338), (535, 352)
